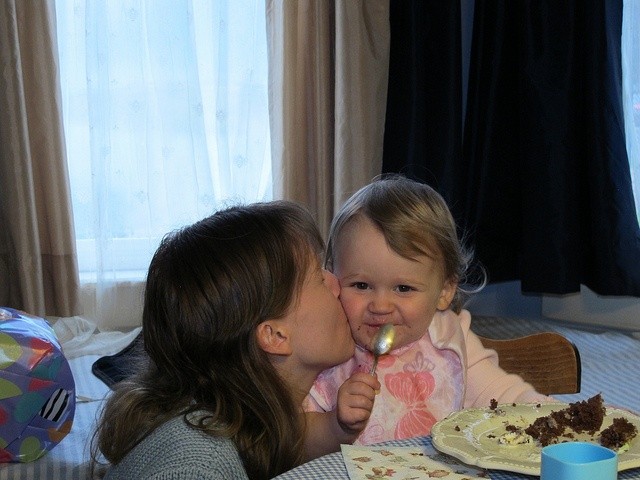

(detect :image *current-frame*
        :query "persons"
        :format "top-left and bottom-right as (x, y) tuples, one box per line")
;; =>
(293, 173), (564, 466)
(87, 199), (356, 480)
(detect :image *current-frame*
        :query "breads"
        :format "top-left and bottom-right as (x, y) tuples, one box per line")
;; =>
(600, 417), (638, 456)
(498, 392), (603, 449)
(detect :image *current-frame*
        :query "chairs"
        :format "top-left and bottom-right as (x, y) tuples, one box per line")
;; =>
(471, 332), (580, 398)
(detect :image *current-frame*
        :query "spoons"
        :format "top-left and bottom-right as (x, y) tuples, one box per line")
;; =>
(369, 323), (396, 376)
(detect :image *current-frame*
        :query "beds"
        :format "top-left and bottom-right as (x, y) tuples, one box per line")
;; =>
(0, 310), (640, 479)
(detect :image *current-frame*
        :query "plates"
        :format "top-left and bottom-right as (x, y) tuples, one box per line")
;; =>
(430, 401), (640, 476)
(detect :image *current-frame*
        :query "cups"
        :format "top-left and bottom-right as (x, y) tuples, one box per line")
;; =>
(540, 441), (618, 480)
